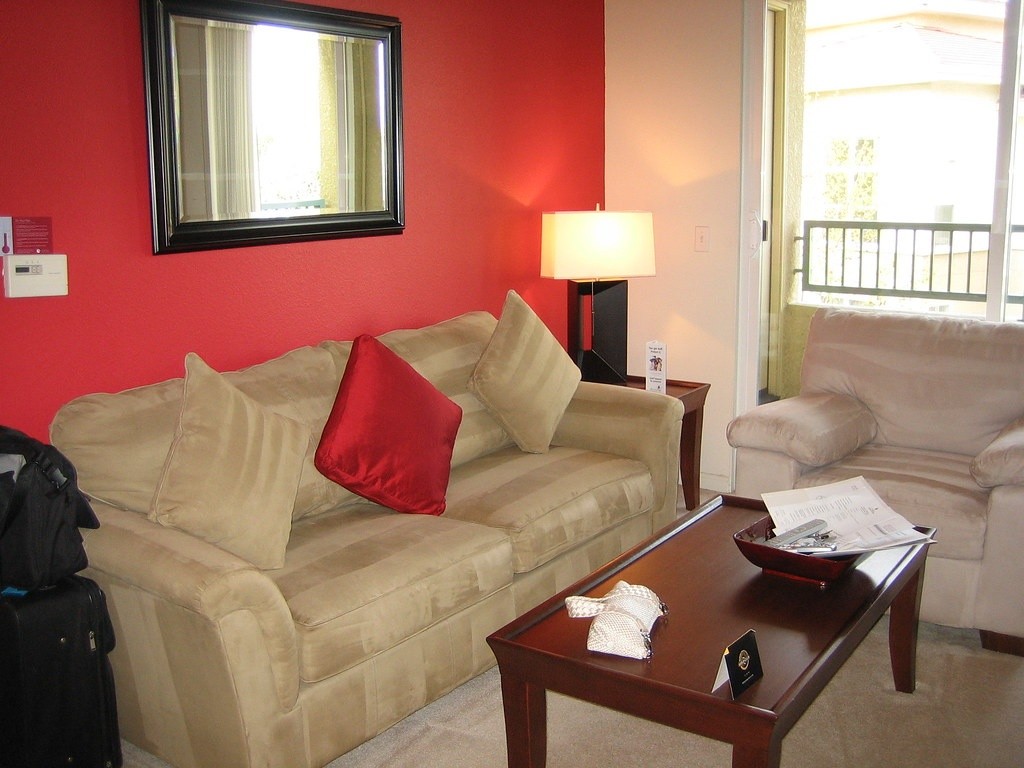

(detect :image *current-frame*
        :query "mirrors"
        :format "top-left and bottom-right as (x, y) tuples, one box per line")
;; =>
(140, 0), (406, 257)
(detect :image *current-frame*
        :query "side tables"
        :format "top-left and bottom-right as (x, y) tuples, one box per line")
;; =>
(626, 373), (711, 511)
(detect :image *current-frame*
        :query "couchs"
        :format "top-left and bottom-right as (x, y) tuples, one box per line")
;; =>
(725, 306), (1024, 656)
(49, 311), (684, 768)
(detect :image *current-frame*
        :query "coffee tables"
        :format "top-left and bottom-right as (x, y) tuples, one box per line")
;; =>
(486, 494), (939, 768)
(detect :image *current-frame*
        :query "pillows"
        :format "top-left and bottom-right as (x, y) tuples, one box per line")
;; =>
(466, 289), (582, 454)
(149, 353), (312, 571)
(314, 333), (463, 517)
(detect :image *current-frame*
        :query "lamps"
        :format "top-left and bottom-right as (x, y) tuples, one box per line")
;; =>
(540, 203), (657, 387)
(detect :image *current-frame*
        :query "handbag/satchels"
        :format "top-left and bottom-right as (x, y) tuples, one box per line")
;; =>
(566, 581), (668, 664)
(0, 423), (99, 589)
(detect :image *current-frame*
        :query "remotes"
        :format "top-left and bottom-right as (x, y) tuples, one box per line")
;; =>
(784, 545), (838, 552)
(764, 519), (827, 548)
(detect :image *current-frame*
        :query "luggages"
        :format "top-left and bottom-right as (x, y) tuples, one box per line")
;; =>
(1, 573), (126, 768)
